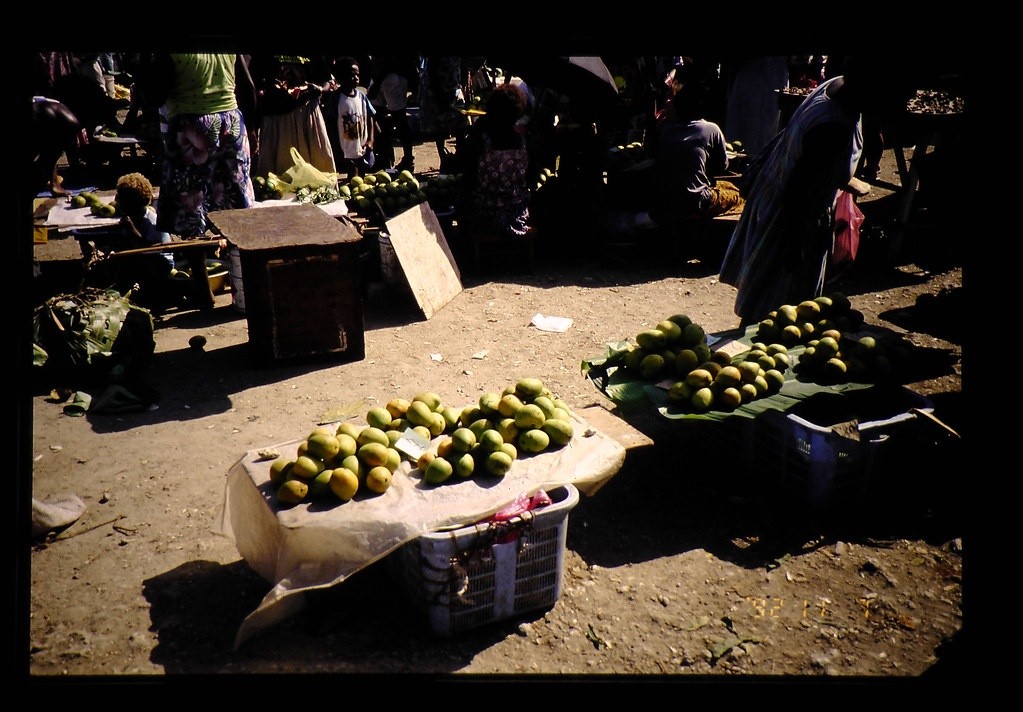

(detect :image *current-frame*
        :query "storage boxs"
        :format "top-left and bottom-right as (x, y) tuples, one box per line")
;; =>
(240, 248), (364, 372)
(377, 231), (398, 277)
(435, 206), (456, 241)
(393, 483), (579, 637)
(774, 384), (934, 498)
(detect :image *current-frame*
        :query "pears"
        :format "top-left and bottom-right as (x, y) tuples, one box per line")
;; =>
(252, 176), (283, 201)
(338, 169), (461, 216)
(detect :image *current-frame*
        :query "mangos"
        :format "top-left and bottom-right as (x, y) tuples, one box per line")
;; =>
(270, 379), (575, 502)
(627, 292), (882, 408)
(70, 192), (117, 217)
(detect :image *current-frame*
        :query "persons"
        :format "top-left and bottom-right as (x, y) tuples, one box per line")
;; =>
(723, 54), (793, 156)
(719, 71), (891, 324)
(642, 87), (741, 223)
(32, 51), (536, 312)
